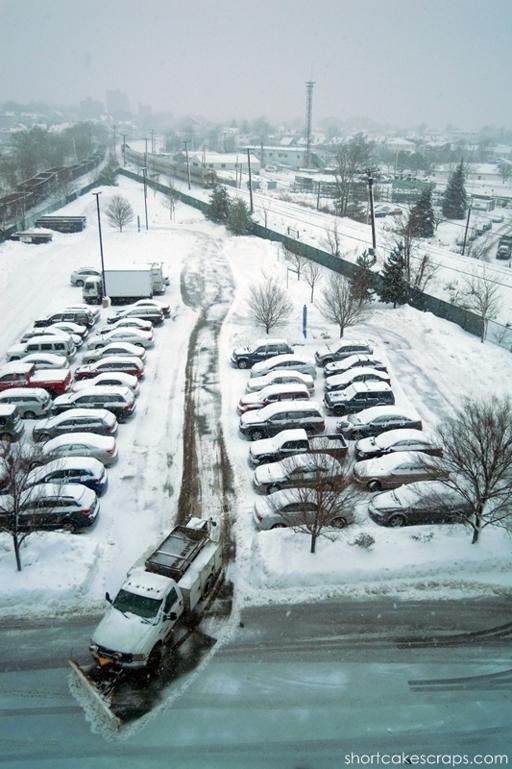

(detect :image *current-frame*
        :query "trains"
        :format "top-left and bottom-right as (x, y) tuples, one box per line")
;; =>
(0, 142), (108, 221)
(120, 143), (216, 188)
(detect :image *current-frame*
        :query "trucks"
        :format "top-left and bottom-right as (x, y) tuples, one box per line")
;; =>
(82, 268), (156, 304)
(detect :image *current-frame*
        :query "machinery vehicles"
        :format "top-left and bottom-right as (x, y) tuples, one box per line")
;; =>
(59, 517), (228, 736)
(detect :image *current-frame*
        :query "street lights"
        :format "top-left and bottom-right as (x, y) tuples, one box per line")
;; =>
(91, 191), (107, 298)
(142, 165), (150, 230)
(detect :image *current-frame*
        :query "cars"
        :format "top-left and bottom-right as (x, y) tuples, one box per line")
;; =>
(334, 197), (403, 219)
(71, 267), (103, 288)
(457, 215), (511, 259)
(377, 172), (431, 184)
